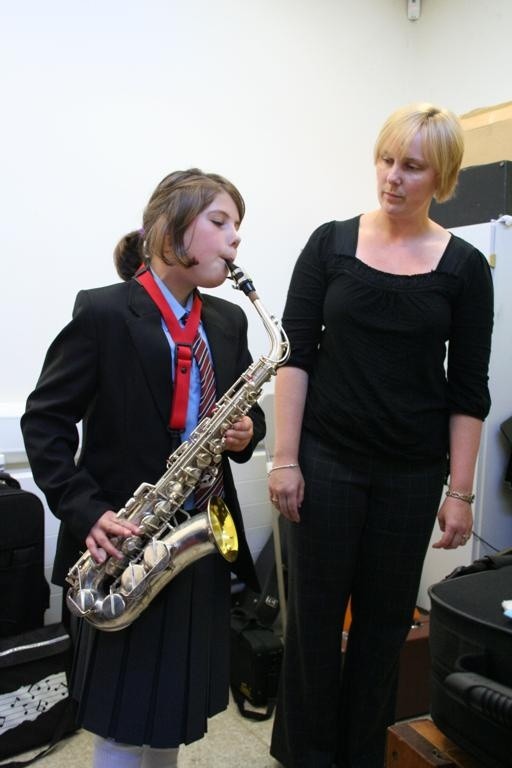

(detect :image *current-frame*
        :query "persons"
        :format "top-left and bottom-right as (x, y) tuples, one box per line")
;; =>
(268, 102), (498, 767)
(20, 167), (267, 768)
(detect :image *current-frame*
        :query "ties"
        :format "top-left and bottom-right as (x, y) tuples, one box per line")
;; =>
(181, 312), (226, 512)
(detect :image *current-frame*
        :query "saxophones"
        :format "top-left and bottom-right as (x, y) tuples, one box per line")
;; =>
(67, 261), (292, 633)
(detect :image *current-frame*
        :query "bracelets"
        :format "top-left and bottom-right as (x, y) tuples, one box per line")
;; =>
(269, 463), (298, 474)
(445, 491), (476, 505)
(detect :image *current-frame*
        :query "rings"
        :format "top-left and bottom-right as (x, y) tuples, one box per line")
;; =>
(463, 534), (471, 540)
(271, 497), (277, 504)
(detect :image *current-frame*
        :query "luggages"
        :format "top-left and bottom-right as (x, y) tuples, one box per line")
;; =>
(423, 549), (512, 766)
(0, 471), (50, 636)
